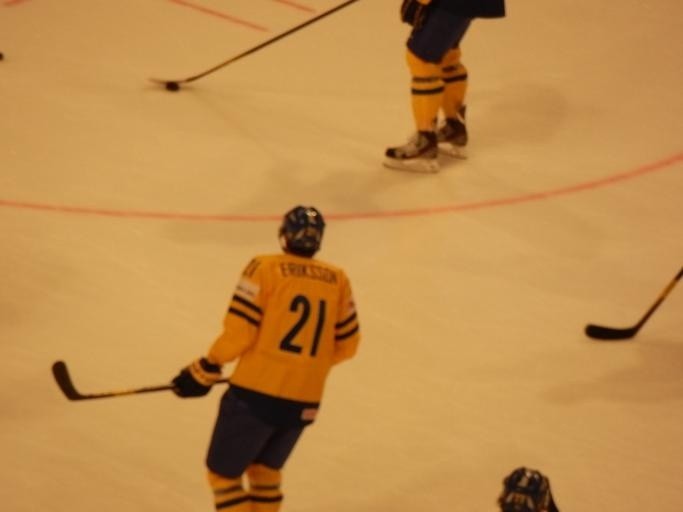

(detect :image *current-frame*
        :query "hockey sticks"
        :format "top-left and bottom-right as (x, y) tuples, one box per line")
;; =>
(52, 361), (234, 401)
(149, 0), (359, 84)
(585, 270), (683, 340)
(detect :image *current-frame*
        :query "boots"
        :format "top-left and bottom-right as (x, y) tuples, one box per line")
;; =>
(438, 106), (468, 147)
(385, 119), (439, 160)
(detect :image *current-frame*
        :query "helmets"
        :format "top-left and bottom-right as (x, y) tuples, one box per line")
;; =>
(277, 205), (325, 258)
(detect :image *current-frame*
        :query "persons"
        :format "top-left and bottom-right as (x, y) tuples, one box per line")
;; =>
(173, 203), (359, 512)
(381, 1), (505, 172)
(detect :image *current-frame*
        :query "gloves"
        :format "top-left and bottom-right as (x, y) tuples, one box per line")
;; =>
(170, 358), (222, 398)
